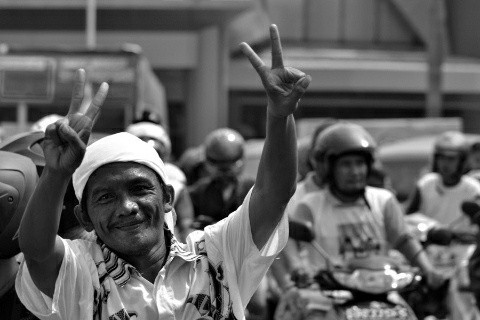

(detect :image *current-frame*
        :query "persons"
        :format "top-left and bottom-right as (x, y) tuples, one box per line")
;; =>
(30, 109), (480, 320)
(14, 22), (313, 320)
(280, 123), (443, 300)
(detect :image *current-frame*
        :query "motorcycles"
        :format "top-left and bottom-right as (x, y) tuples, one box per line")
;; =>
(285, 201), (480, 320)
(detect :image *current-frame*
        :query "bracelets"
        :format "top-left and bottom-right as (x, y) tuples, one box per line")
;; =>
(410, 248), (424, 262)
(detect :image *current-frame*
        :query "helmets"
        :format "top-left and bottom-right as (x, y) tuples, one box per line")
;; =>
(206, 127), (246, 163)
(313, 121), (376, 185)
(429, 130), (474, 175)
